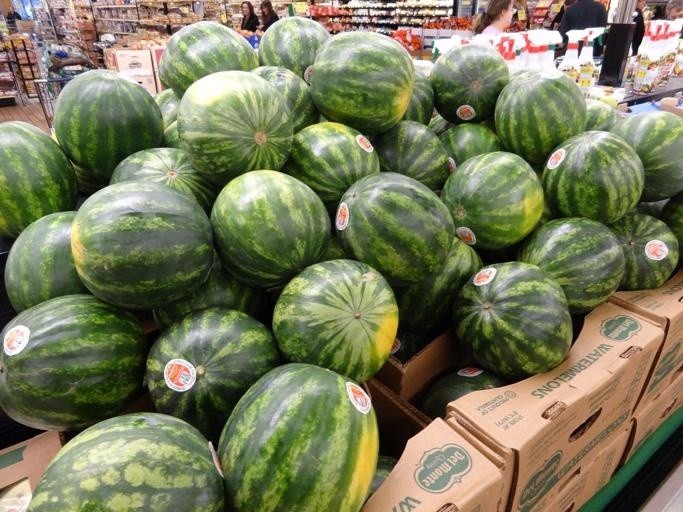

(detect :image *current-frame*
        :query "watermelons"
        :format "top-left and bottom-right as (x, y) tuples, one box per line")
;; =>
(0, 16), (683, 511)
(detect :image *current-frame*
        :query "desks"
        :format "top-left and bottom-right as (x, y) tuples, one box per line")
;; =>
(618, 74), (683, 105)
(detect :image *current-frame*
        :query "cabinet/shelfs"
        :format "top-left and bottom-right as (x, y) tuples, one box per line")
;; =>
(1, 0), (562, 104)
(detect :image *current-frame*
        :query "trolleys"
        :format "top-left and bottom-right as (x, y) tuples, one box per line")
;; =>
(33, 76), (76, 133)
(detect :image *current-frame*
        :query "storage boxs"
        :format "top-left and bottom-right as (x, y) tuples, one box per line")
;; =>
(0, 430), (68, 512)
(360, 268), (682, 511)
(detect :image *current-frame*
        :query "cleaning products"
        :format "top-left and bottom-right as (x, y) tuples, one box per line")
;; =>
(494, 20), (683, 97)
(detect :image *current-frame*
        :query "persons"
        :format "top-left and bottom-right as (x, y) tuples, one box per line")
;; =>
(550, 0), (577, 30)
(474, 0), (514, 34)
(260, 0), (279, 32)
(560, 0), (607, 57)
(7, 6), (22, 29)
(0, 9), (5, 22)
(632, 1), (646, 57)
(241, 1), (259, 31)
(666, 0), (683, 39)
